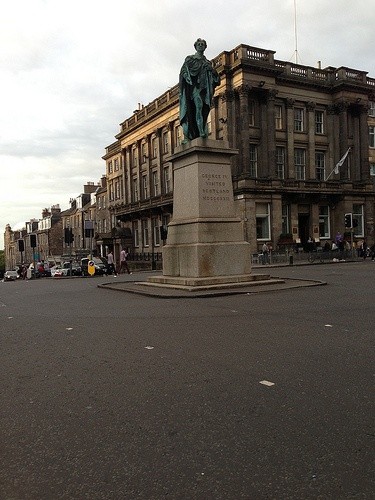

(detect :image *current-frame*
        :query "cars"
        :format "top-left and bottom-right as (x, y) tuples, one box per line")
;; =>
(3, 256), (114, 282)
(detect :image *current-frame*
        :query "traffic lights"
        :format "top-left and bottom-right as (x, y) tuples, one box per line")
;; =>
(29, 234), (37, 247)
(353, 219), (358, 228)
(345, 213), (352, 229)
(18, 240), (24, 251)
(65, 228), (74, 243)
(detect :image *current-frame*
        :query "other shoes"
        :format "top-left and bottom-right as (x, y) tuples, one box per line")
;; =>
(129, 273), (132, 275)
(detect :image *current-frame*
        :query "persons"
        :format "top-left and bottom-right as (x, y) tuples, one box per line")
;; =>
(177, 38), (220, 144)
(324, 232), (375, 262)
(118, 248), (132, 276)
(262, 242), (269, 256)
(17, 260), (45, 280)
(103, 250), (118, 277)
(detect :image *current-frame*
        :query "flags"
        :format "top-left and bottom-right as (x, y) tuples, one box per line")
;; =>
(334, 148), (349, 175)
